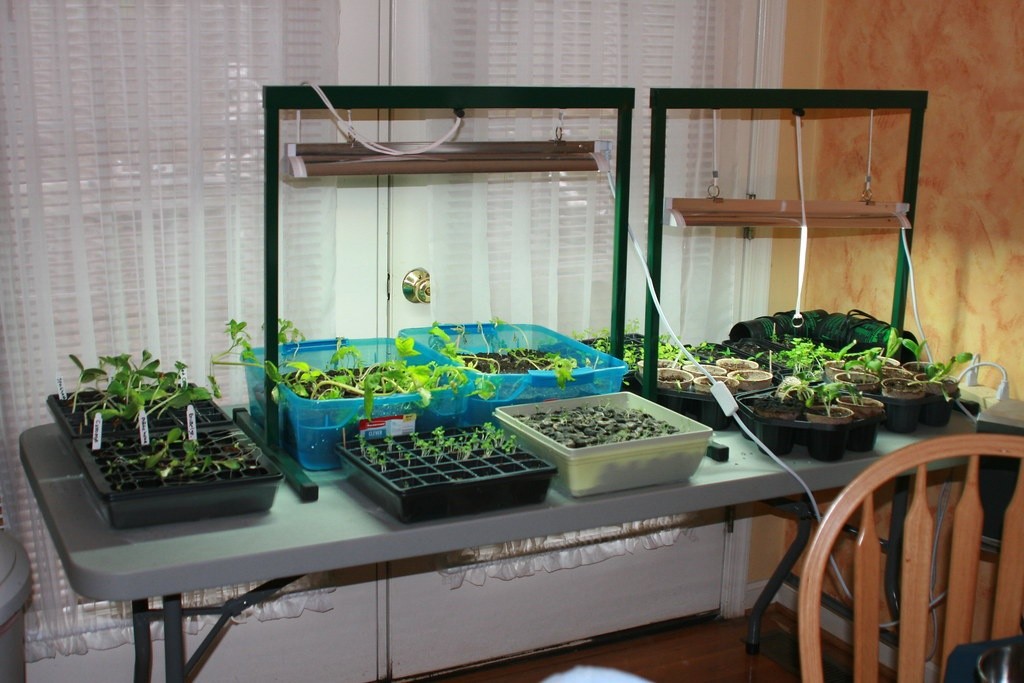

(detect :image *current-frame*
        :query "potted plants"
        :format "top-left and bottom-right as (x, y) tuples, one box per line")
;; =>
(804, 380), (856, 424)
(46, 348), (232, 473)
(900, 339), (936, 374)
(880, 377), (954, 402)
(492, 391), (713, 501)
(727, 367), (773, 391)
(753, 382), (814, 421)
(209, 318), (473, 472)
(834, 350), (884, 392)
(71, 424), (285, 532)
(335, 421), (558, 525)
(858, 326), (903, 373)
(397, 315), (628, 430)
(836, 383), (884, 417)
(913, 352), (973, 395)
(825, 339), (882, 381)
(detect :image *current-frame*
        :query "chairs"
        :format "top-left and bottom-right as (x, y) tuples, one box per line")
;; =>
(799, 433), (1024, 683)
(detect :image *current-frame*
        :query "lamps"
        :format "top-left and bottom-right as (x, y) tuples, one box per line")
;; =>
(663, 110), (912, 228)
(279, 110), (615, 183)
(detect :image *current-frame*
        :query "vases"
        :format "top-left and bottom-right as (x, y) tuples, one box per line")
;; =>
(636, 359), (681, 378)
(716, 358), (759, 372)
(692, 375), (741, 395)
(657, 367), (695, 392)
(682, 364), (728, 378)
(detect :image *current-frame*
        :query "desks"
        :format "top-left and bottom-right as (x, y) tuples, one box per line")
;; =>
(17, 400), (977, 683)
